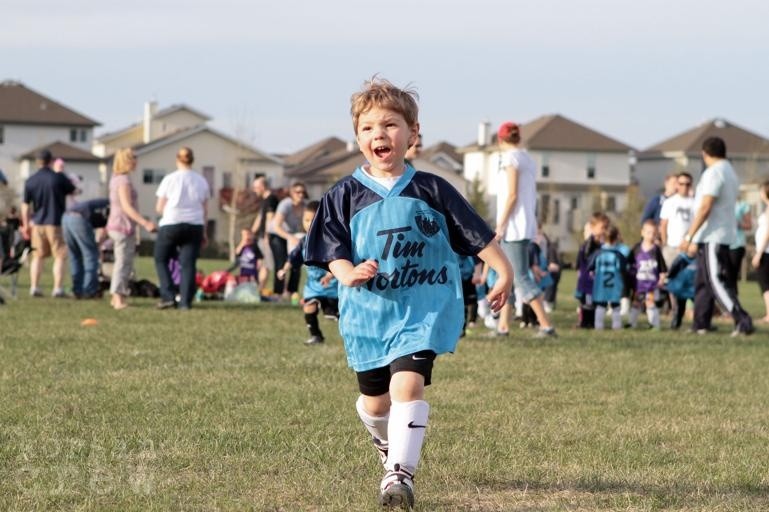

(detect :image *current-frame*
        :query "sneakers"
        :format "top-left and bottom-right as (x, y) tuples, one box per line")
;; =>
(372, 435), (388, 471)
(380, 463), (415, 512)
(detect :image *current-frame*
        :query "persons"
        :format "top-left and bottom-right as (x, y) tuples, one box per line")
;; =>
(404, 135), (423, 170)
(736, 179), (769, 322)
(679, 136), (756, 339)
(52, 156), (84, 210)
(20, 149), (84, 298)
(495, 124), (558, 341)
(303, 79), (514, 512)
(154, 147), (210, 309)
(462, 223), (561, 338)
(1, 207), (33, 278)
(60, 199), (110, 301)
(105, 148), (156, 311)
(574, 173), (718, 334)
(234, 174), (343, 347)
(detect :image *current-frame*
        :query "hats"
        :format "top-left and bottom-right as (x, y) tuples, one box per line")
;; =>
(497, 122), (519, 138)
(37, 149), (50, 160)
